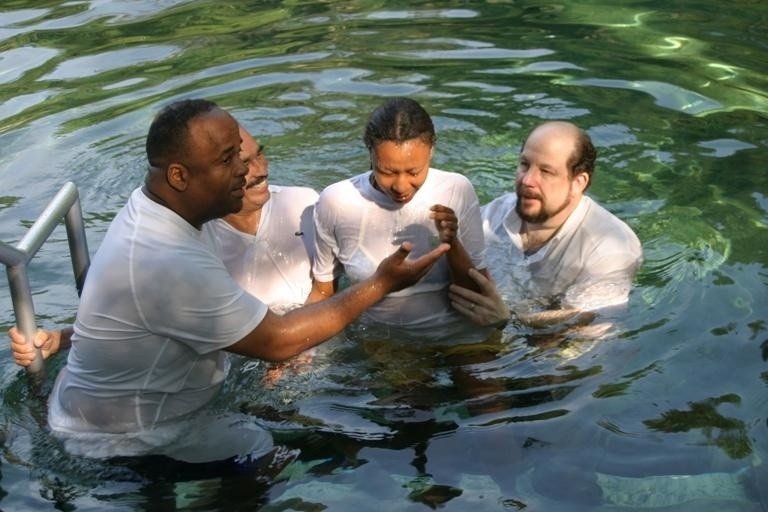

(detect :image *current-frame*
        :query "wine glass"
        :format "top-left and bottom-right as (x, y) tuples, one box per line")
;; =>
(470, 303), (475, 311)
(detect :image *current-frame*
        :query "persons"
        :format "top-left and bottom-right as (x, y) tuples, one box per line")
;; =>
(7, 127), (318, 367)
(46, 100), (452, 512)
(264, 96), (512, 468)
(447, 120), (644, 422)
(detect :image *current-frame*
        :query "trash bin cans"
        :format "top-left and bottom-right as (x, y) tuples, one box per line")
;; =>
(505, 311), (521, 328)
